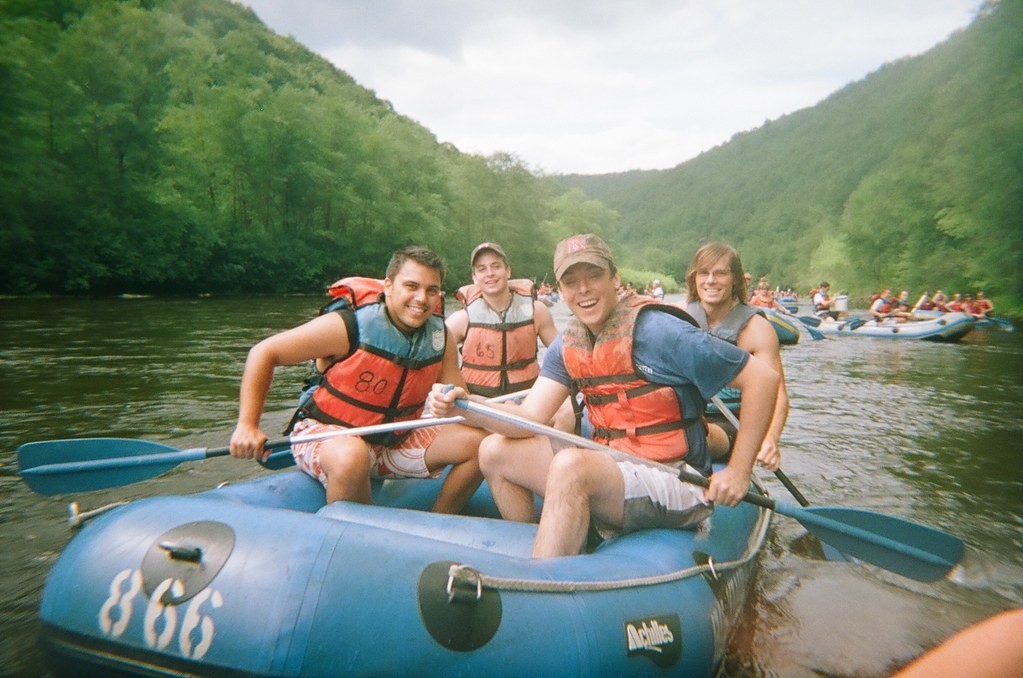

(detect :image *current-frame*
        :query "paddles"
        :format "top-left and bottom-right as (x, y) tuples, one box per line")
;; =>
(773, 308), (822, 328)
(789, 313), (826, 340)
(16, 412), (470, 498)
(838, 310), (900, 332)
(443, 383), (964, 583)
(972, 315), (1014, 333)
(711, 393), (857, 562)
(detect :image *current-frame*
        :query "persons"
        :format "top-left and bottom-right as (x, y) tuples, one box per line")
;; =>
(671, 242), (790, 473)
(539, 282), (554, 295)
(769, 288), (798, 300)
(429, 233), (782, 560)
(616, 282), (638, 300)
(871, 288), (994, 323)
(743, 274), (791, 317)
(230, 247), (486, 514)
(812, 281), (848, 330)
(445, 242), (576, 437)
(644, 279), (664, 301)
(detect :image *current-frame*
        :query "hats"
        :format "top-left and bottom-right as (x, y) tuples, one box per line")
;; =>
(554, 233), (614, 280)
(470, 242), (505, 267)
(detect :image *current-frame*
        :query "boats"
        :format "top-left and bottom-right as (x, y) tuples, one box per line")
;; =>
(777, 298), (798, 313)
(38, 410), (772, 678)
(890, 610), (1022, 677)
(538, 294), (559, 306)
(913, 309), (988, 325)
(803, 311), (975, 341)
(754, 306), (799, 344)
